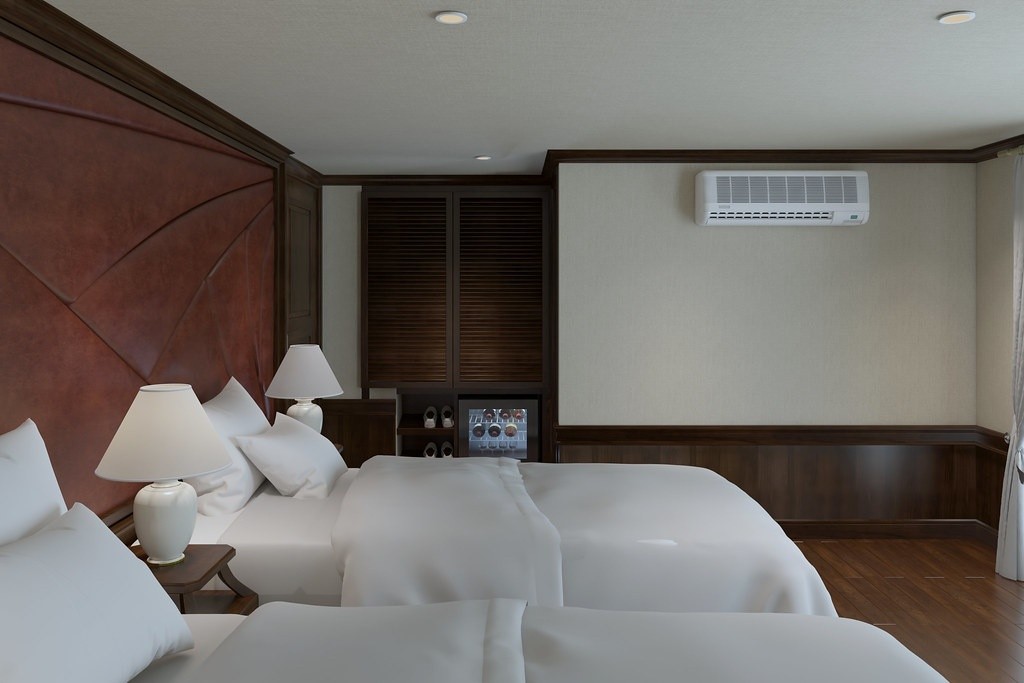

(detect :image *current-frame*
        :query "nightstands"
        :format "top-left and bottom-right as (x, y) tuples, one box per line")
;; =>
(128, 544), (259, 618)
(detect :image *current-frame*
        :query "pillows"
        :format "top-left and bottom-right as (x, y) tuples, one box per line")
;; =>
(182, 375), (272, 516)
(0, 503), (195, 683)
(0, 418), (70, 547)
(237, 413), (349, 500)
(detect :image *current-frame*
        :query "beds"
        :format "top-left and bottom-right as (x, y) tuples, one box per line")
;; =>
(193, 454), (842, 617)
(130, 600), (949, 683)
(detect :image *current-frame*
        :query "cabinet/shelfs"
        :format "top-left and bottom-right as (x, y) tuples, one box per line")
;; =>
(397, 389), (554, 463)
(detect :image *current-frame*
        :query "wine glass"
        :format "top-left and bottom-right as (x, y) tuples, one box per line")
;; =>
(499, 441), (507, 456)
(478, 440), (487, 456)
(509, 441), (518, 456)
(488, 441), (497, 456)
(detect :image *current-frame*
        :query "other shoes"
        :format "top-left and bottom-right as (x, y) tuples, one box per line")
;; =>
(441, 440), (454, 458)
(423, 441), (439, 459)
(442, 405), (455, 427)
(423, 406), (439, 428)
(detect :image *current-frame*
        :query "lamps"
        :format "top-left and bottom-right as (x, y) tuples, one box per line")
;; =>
(95, 382), (226, 567)
(265, 344), (344, 434)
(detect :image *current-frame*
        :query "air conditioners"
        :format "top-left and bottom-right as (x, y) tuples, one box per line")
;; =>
(694, 169), (870, 227)
